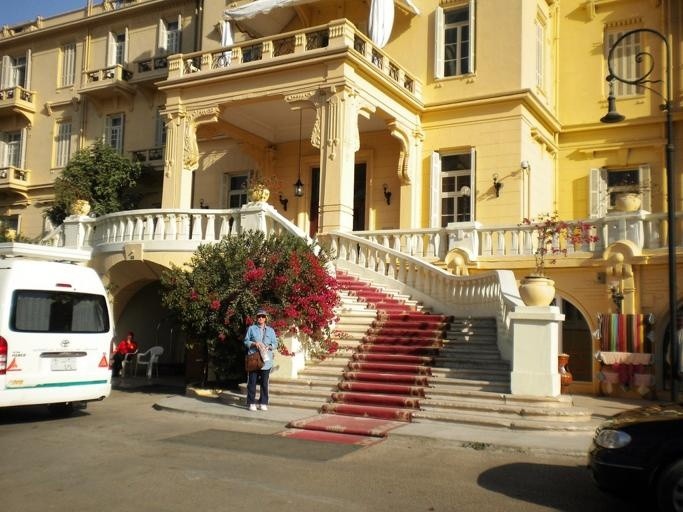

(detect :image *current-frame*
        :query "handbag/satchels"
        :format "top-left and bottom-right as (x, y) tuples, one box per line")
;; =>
(245, 351), (264, 371)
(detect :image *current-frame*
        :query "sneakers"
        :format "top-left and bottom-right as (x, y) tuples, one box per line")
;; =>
(249, 404), (257, 410)
(260, 404), (267, 410)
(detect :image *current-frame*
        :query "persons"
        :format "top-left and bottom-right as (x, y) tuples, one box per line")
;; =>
(112, 331), (137, 377)
(244, 310), (278, 410)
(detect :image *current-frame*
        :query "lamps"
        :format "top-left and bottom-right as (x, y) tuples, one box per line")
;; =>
(293, 109), (306, 197)
(492, 173), (503, 196)
(382, 183), (392, 205)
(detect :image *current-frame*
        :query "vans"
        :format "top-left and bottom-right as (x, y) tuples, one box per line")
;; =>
(0, 241), (115, 408)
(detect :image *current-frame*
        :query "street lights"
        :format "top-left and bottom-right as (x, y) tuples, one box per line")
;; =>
(600, 27), (677, 402)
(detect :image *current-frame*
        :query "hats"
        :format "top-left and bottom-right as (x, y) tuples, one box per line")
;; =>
(257, 310), (267, 315)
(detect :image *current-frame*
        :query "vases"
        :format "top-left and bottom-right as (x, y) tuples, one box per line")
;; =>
(247, 185), (269, 203)
(520, 279), (555, 306)
(69, 200), (90, 215)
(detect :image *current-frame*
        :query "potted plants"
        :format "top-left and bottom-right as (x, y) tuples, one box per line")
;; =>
(597, 181), (659, 211)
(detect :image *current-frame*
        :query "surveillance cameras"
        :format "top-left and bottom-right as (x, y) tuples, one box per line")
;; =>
(521, 161), (528, 169)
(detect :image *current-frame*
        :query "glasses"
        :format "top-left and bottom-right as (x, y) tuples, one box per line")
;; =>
(258, 315), (267, 318)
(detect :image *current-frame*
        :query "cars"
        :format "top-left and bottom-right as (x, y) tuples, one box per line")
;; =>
(590, 405), (680, 512)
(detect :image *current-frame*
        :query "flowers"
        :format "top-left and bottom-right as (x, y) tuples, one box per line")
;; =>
(515, 213), (598, 278)
(240, 173), (283, 203)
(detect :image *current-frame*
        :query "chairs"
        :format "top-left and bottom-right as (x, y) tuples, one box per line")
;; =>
(110, 345), (165, 379)
